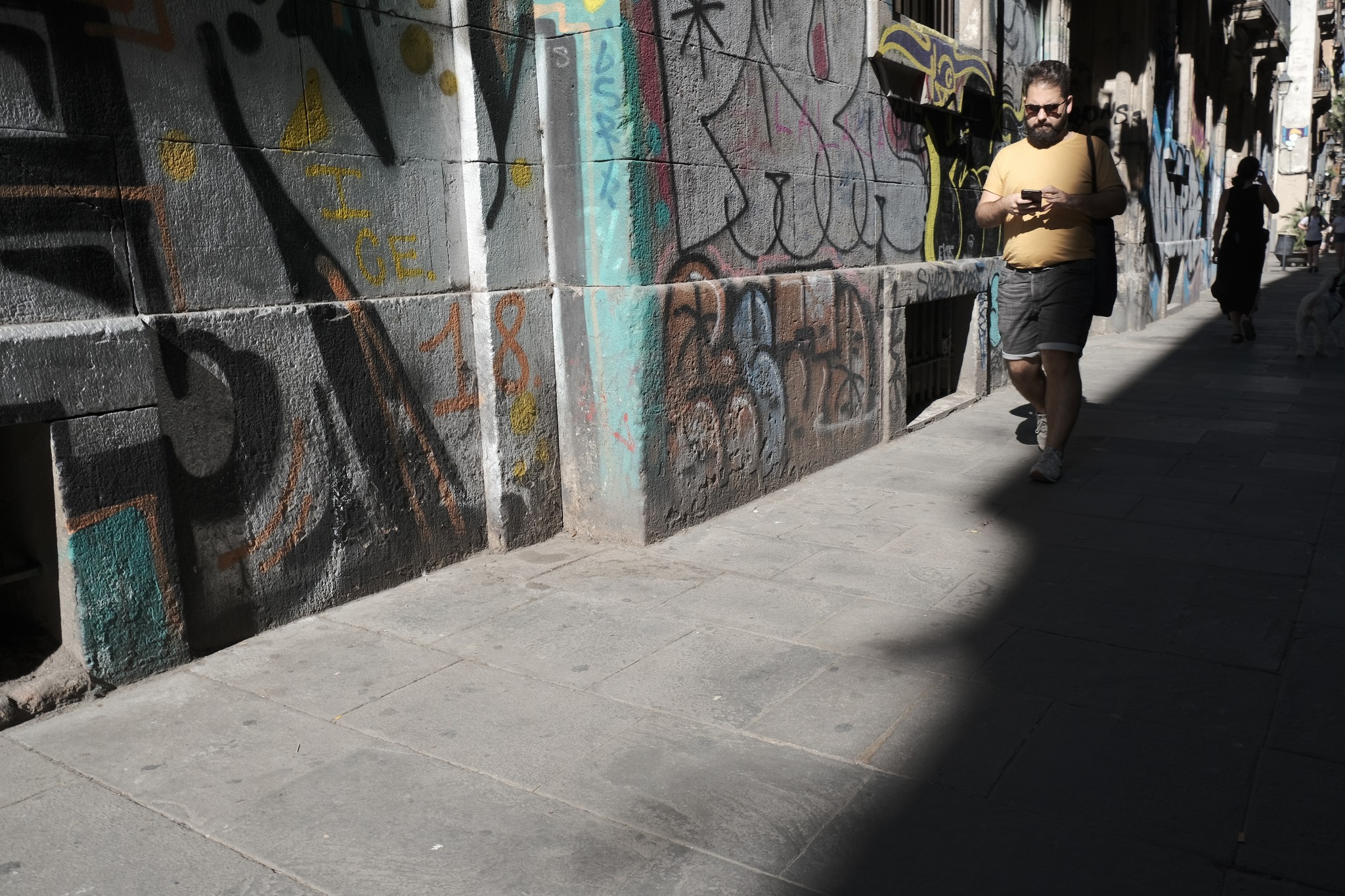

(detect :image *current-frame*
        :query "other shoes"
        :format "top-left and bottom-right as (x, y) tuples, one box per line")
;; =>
(1240, 314), (1256, 341)
(1307, 268), (1312, 272)
(1232, 333), (1243, 343)
(1314, 266), (1318, 272)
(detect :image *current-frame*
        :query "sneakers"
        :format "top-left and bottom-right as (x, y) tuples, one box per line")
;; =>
(1035, 410), (1048, 451)
(1029, 446), (1063, 483)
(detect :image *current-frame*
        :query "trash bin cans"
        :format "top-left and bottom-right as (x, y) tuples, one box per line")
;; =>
(1274, 234), (1297, 258)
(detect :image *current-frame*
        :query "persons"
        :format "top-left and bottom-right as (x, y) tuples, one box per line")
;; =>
(1298, 205), (1329, 272)
(1330, 207), (1345, 270)
(1209, 156), (1279, 345)
(975, 59), (1127, 484)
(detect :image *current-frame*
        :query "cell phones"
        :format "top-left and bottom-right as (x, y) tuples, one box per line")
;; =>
(1021, 189), (1042, 209)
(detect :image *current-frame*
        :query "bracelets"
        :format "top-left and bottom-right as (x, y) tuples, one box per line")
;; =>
(1213, 247), (1220, 251)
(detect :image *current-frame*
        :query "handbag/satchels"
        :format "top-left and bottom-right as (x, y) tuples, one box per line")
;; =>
(1093, 217), (1118, 317)
